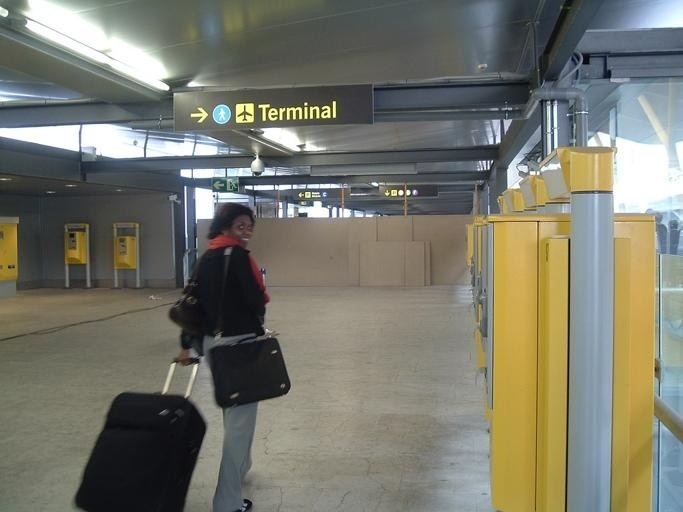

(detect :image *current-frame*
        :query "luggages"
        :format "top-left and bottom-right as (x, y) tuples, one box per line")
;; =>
(74, 357), (207, 512)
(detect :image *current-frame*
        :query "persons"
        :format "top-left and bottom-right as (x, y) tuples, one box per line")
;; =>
(170, 201), (269, 512)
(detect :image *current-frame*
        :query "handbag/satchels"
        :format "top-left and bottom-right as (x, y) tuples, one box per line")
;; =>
(169, 253), (208, 332)
(207, 335), (292, 409)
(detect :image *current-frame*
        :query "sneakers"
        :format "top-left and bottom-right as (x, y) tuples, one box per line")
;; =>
(233, 498), (253, 512)
